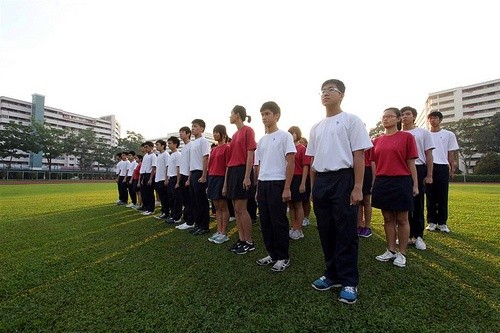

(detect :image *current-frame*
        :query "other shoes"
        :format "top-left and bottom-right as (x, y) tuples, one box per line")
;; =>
(117, 201), (148, 212)
(189, 225), (210, 236)
(155, 201), (162, 206)
(174, 222), (196, 229)
(229, 217), (236, 221)
(154, 211), (169, 220)
(210, 213), (216, 217)
(140, 210), (155, 216)
(164, 217), (181, 223)
(252, 220), (257, 225)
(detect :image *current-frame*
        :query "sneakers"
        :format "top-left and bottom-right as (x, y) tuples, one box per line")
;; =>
(396, 237), (414, 245)
(437, 224), (450, 233)
(338, 286), (358, 304)
(426, 223), (437, 231)
(271, 258), (290, 272)
(375, 249), (397, 262)
(256, 255), (277, 265)
(289, 227), (304, 239)
(207, 232), (230, 243)
(229, 239), (256, 254)
(414, 236), (426, 250)
(357, 226), (372, 237)
(393, 252), (407, 267)
(302, 217), (310, 226)
(311, 275), (342, 290)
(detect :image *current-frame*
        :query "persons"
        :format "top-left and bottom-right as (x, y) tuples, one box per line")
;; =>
(426, 111), (458, 232)
(358, 150), (371, 237)
(396, 106), (436, 251)
(210, 138), (258, 222)
(369, 107), (419, 267)
(207, 125), (231, 244)
(186, 119), (210, 236)
(305, 79), (374, 303)
(226, 105), (257, 255)
(115, 126), (195, 230)
(253, 101), (298, 271)
(287, 126), (312, 239)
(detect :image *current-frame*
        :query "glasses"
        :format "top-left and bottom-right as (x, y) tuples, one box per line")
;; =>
(382, 115), (398, 118)
(319, 87), (342, 94)
